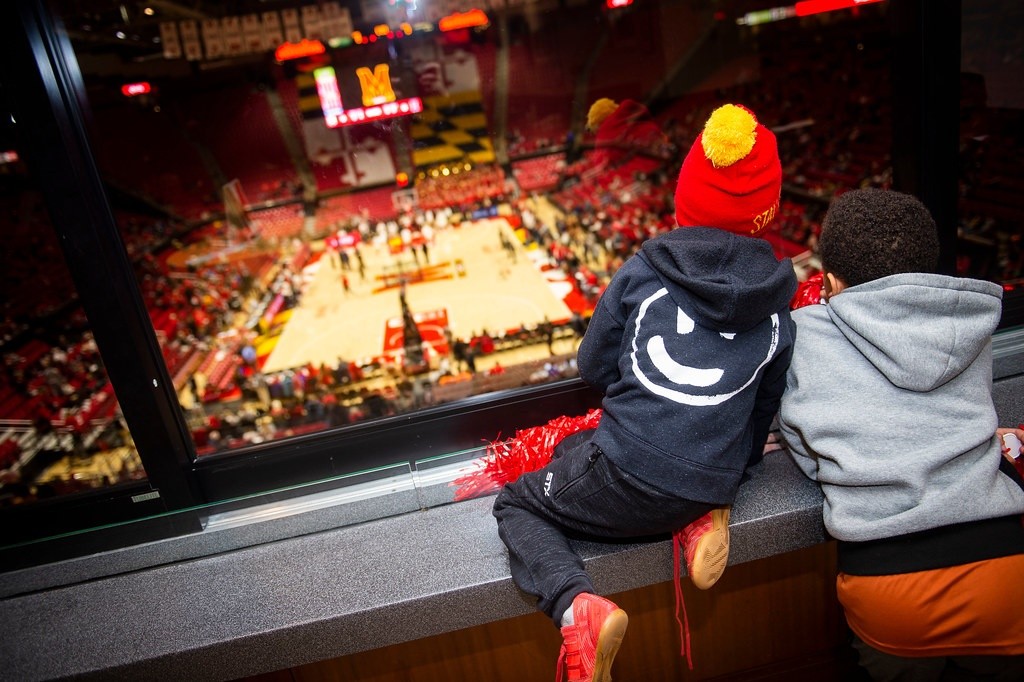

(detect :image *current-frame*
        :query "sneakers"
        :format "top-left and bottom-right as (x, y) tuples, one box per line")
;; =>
(671, 508), (730, 671)
(556, 591), (628, 682)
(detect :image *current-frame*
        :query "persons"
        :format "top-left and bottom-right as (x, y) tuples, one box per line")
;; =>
(492, 104), (796, 681)
(0, 160), (683, 503)
(777, 187), (1023, 682)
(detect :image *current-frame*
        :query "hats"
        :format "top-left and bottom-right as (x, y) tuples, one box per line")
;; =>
(675, 103), (781, 239)
